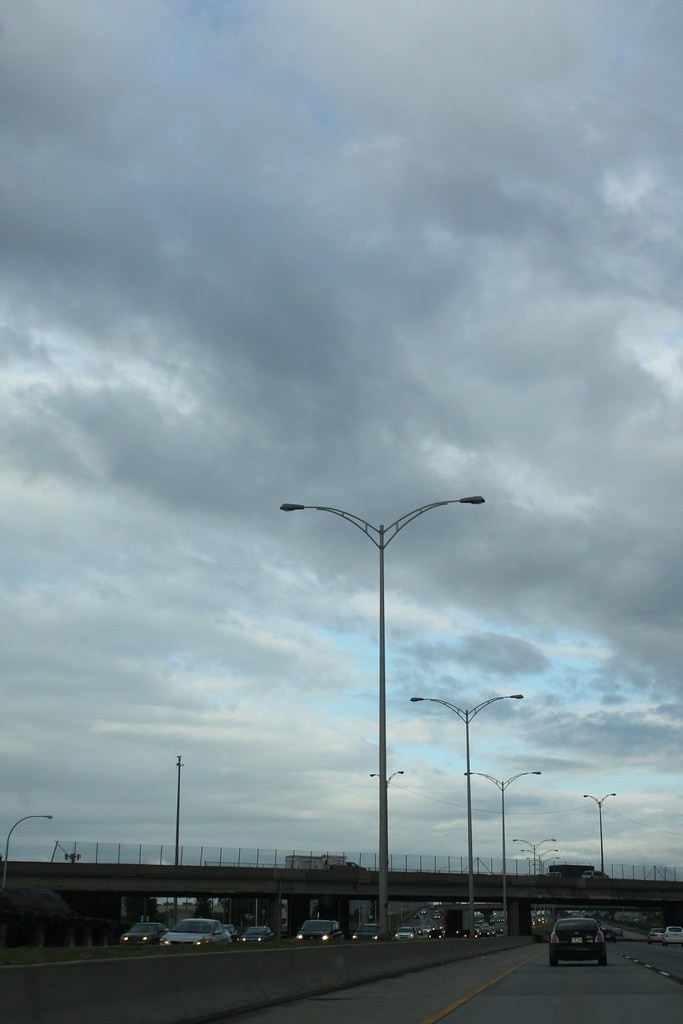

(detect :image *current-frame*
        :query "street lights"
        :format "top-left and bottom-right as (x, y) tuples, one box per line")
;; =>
(463, 771), (542, 936)
(279, 495), (486, 942)
(512, 838), (560, 864)
(2, 814), (53, 889)
(410, 694), (524, 940)
(583, 793), (617, 873)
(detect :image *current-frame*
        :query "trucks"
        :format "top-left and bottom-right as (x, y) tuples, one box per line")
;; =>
(547, 865), (594, 878)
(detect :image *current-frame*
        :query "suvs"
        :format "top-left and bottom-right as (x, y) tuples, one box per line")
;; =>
(581, 869), (611, 881)
(603, 928), (617, 943)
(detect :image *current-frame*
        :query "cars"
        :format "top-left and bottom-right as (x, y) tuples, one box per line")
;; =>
(118, 908), (549, 946)
(549, 918), (608, 967)
(613, 927), (624, 937)
(663, 926), (683, 946)
(647, 927), (666, 944)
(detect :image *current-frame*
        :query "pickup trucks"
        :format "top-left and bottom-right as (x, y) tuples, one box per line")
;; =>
(327, 862), (367, 872)
